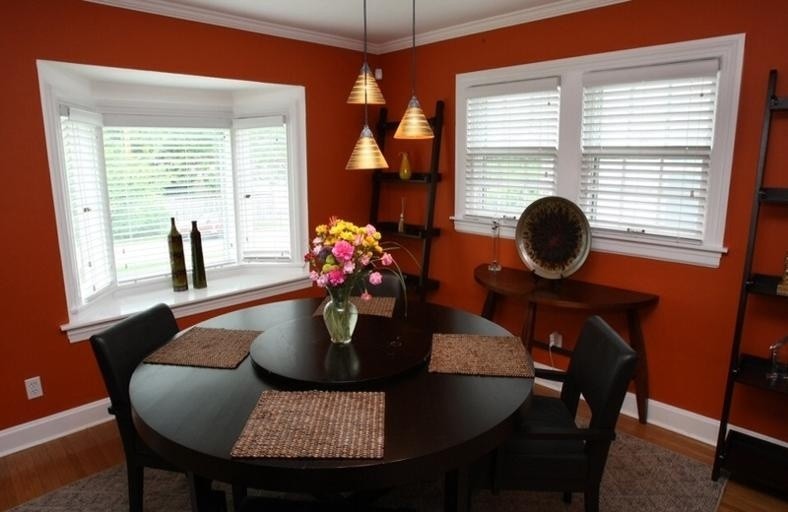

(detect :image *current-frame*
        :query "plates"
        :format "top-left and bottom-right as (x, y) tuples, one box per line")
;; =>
(514, 195), (592, 281)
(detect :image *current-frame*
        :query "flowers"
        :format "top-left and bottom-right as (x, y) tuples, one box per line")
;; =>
(302, 215), (421, 339)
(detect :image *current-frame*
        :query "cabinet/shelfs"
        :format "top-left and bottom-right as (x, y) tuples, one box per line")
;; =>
(366, 100), (446, 303)
(710, 69), (788, 502)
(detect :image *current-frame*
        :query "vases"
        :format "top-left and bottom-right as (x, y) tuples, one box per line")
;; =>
(398, 152), (412, 181)
(488, 217), (502, 271)
(167, 217), (188, 292)
(322, 285), (359, 345)
(190, 220), (208, 289)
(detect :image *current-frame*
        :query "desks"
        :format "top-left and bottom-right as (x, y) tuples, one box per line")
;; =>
(473, 263), (658, 424)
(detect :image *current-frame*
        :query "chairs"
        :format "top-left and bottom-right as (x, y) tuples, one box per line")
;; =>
(338, 268), (408, 301)
(232, 483), (394, 512)
(463, 314), (639, 511)
(88, 303), (222, 512)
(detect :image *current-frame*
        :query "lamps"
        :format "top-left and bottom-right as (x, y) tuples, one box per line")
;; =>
(344, 1), (388, 172)
(392, 1), (438, 143)
(344, 1), (387, 106)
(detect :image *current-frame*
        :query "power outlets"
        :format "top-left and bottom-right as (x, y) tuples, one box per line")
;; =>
(24, 376), (44, 400)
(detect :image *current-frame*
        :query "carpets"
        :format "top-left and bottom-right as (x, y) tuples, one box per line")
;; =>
(0, 414), (726, 511)
